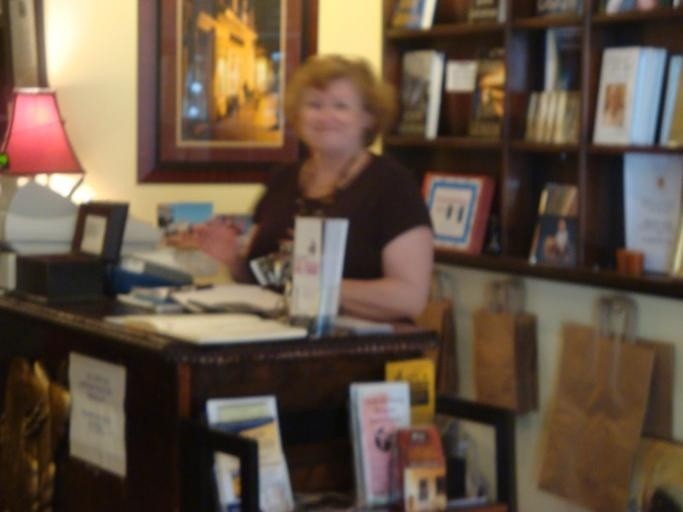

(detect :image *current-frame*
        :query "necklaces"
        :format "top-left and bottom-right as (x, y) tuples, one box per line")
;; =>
(291, 151), (363, 217)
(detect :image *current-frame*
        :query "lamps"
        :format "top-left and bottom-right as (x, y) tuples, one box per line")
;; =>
(0, 86), (85, 194)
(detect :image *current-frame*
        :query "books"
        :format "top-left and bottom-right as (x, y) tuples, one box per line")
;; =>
(350, 380), (417, 509)
(387, 2), (680, 150)
(214, 454), (296, 511)
(288, 215), (352, 337)
(104, 281), (310, 346)
(208, 393), (285, 456)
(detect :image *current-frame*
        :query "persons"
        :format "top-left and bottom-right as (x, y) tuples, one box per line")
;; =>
(182, 53), (435, 323)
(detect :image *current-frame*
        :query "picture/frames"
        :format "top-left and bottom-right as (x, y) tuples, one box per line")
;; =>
(135, 1), (320, 187)
(433, 394), (520, 512)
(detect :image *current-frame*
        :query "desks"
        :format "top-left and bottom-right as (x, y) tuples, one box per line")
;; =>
(1, 286), (444, 512)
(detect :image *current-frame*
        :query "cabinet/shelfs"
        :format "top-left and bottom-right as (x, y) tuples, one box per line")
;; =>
(378, 1), (683, 300)
